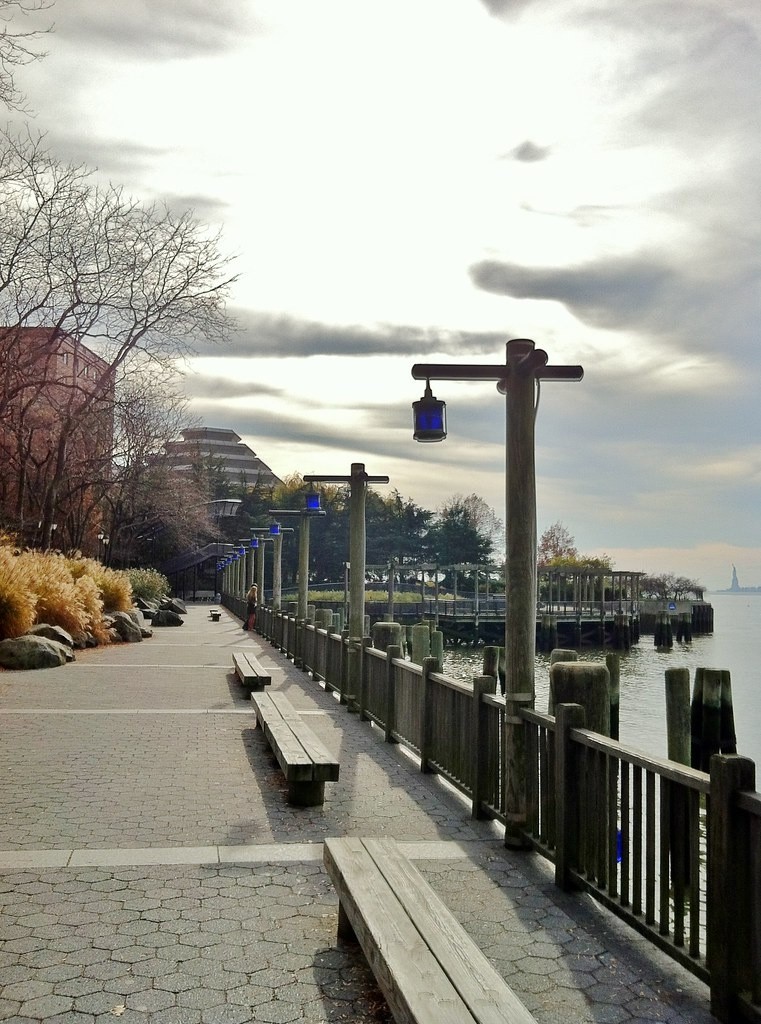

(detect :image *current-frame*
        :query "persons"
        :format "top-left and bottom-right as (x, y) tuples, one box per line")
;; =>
(241, 583), (260, 632)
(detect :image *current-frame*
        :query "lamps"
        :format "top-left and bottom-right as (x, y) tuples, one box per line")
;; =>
(412, 380), (447, 442)
(268, 516), (280, 536)
(250, 533), (258, 547)
(305, 481), (322, 511)
(217, 544), (245, 572)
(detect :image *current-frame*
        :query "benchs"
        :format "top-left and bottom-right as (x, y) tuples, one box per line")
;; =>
(324, 837), (538, 1024)
(251, 691), (340, 806)
(210, 609), (221, 621)
(232, 652), (271, 700)
